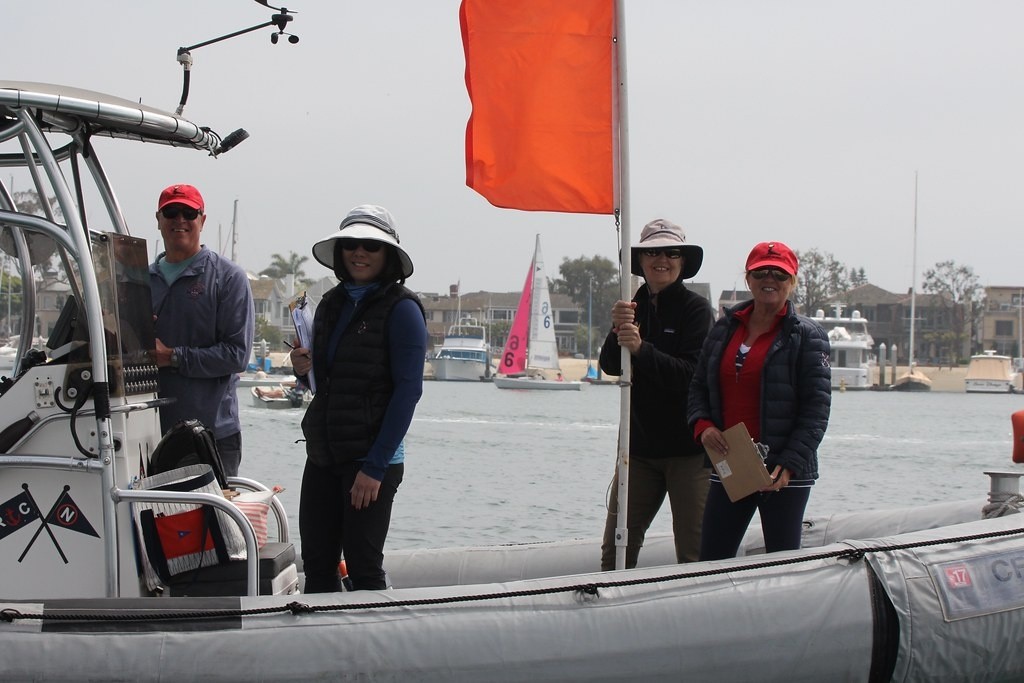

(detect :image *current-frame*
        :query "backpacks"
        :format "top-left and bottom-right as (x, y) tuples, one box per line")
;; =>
(152, 418), (236, 494)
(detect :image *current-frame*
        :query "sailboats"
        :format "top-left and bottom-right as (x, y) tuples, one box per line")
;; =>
(218, 198), (298, 386)
(494, 233), (592, 391)
(891, 168), (933, 392)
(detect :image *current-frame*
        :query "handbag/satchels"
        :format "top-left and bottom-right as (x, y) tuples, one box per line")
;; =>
(231, 485), (286, 552)
(130, 463), (245, 587)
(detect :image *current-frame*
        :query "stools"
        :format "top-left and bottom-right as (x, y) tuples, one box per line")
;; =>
(175, 541), (301, 597)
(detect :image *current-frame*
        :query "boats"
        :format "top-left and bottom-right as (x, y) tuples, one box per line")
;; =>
(425, 277), (498, 381)
(808, 299), (876, 391)
(964, 348), (1019, 393)
(1, 78), (1024, 683)
(250, 383), (314, 409)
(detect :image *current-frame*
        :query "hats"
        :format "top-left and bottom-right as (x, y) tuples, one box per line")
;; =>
(158, 184), (205, 213)
(313, 205), (414, 280)
(620, 219), (703, 279)
(745, 241), (798, 275)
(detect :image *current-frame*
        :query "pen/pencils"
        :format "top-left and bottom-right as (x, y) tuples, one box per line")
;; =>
(283, 340), (311, 360)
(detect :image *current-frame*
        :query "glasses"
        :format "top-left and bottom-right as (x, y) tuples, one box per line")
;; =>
(343, 238), (382, 253)
(162, 205), (199, 220)
(749, 267), (790, 282)
(643, 247), (684, 259)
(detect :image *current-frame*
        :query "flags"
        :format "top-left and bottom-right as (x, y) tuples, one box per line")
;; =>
(460, 0), (622, 215)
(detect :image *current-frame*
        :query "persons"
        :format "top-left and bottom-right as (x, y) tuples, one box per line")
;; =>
(556, 373), (563, 381)
(146, 185), (256, 495)
(601, 219), (717, 572)
(534, 372), (542, 380)
(687, 243), (831, 561)
(290, 205), (427, 593)
